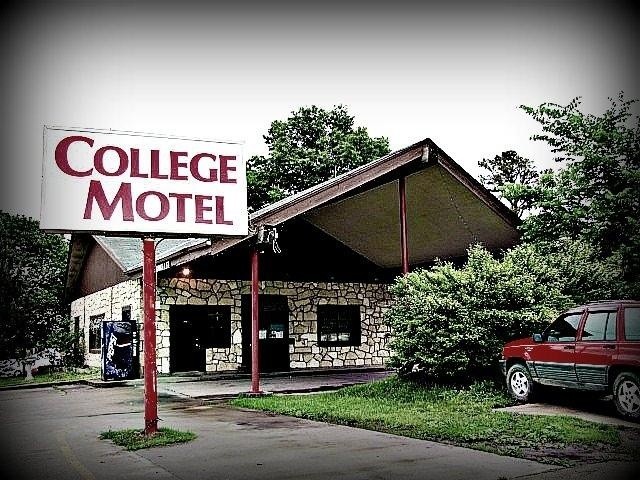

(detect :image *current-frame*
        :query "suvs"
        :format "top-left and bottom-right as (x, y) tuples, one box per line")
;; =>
(499, 299), (640, 422)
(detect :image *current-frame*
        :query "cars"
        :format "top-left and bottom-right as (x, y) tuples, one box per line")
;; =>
(0, 348), (63, 378)
